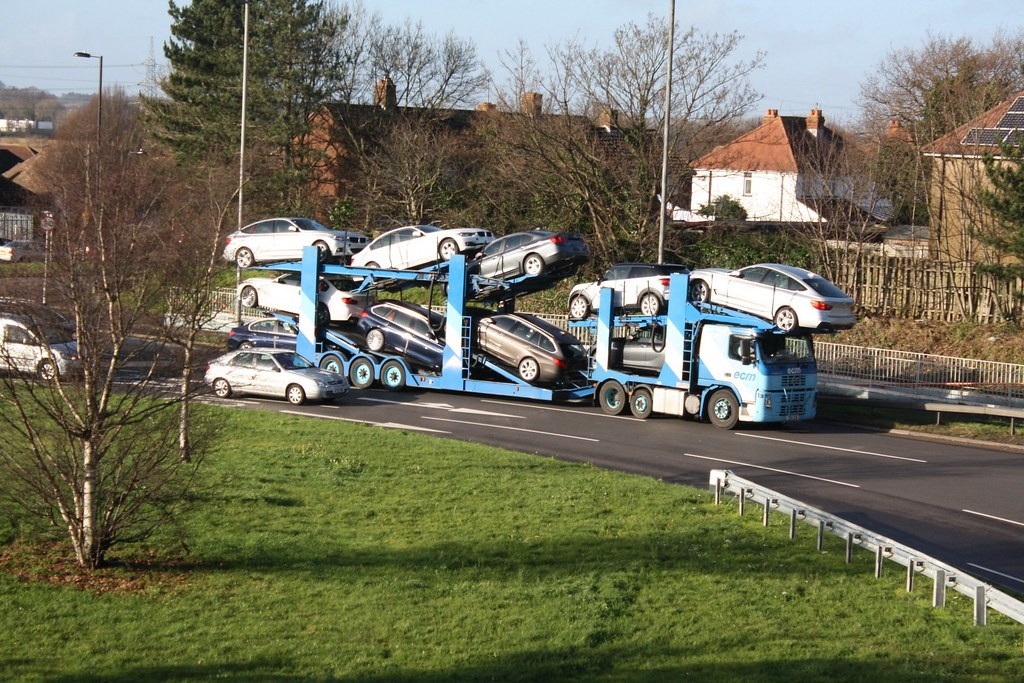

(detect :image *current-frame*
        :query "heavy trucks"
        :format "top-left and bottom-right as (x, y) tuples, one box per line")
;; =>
(591, 273), (821, 430)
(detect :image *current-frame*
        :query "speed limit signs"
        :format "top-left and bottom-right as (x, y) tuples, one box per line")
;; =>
(40, 216), (56, 231)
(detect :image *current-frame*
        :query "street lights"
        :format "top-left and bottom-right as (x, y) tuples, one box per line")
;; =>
(73, 51), (104, 201)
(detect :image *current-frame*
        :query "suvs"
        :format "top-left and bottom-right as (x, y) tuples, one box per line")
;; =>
(567, 261), (692, 314)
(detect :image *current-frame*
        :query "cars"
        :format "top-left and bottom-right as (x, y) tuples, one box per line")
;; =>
(0, 316), (96, 382)
(0, 237), (12, 246)
(221, 217), (372, 270)
(354, 298), (444, 374)
(445, 226), (596, 301)
(235, 272), (378, 326)
(688, 261), (856, 334)
(347, 223), (496, 274)
(222, 316), (299, 352)
(203, 346), (351, 406)
(588, 323), (671, 374)
(464, 304), (590, 386)
(0, 293), (77, 339)
(0, 240), (40, 262)
